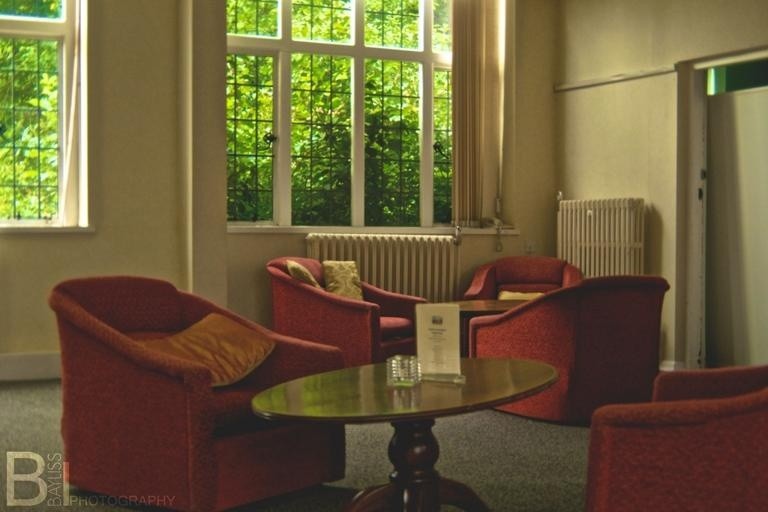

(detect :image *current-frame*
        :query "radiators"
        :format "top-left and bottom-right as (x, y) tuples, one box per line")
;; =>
(307, 232), (461, 305)
(555, 196), (644, 279)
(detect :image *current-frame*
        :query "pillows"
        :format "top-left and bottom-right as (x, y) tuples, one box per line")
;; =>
(136, 313), (276, 388)
(497, 290), (544, 300)
(322, 260), (363, 301)
(286, 260), (320, 288)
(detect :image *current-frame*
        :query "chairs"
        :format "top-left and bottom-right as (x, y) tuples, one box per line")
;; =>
(465, 255), (583, 309)
(468, 275), (671, 425)
(267, 255), (427, 368)
(587, 365), (768, 512)
(49, 276), (344, 512)
